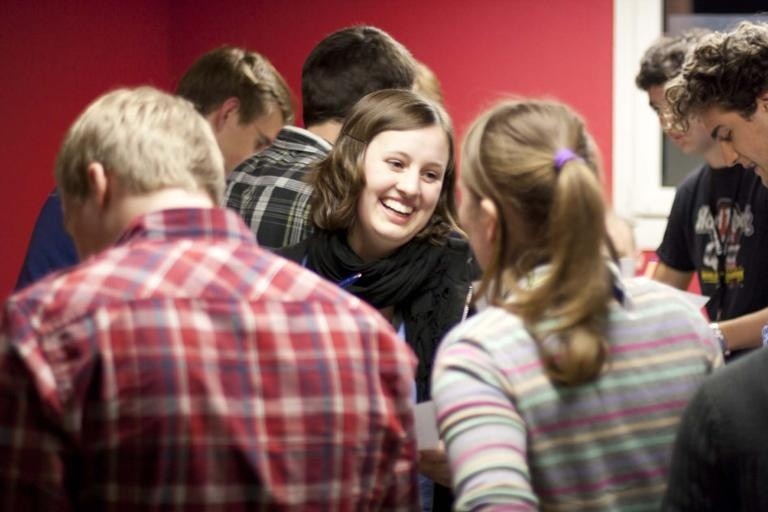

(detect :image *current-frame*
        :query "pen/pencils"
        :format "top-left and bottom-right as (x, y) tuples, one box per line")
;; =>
(460, 286), (473, 322)
(339, 272), (362, 289)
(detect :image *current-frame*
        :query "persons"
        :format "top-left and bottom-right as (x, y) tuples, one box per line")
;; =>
(635, 28), (768, 361)
(654, 327), (767, 510)
(10, 42), (297, 298)
(426, 90), (728, 512)
(413, 60), (443, 108)
(659, 18), (768, 192)
(269, 88), (483, 511)
(0, 81), (422, 512)
(223, 22), (424, 249)
